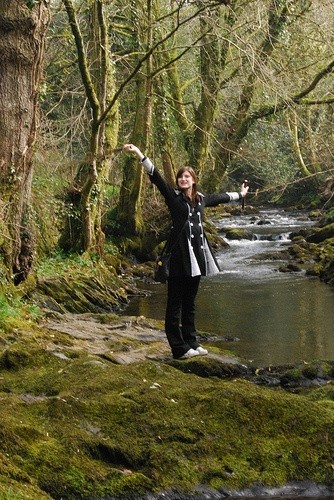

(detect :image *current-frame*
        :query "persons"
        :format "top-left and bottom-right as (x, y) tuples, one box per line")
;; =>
(122, 143), (250, 360)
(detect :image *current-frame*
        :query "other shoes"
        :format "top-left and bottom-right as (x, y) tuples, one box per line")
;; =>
(195, 346), (208, 355)
(179, 348), (199, 359)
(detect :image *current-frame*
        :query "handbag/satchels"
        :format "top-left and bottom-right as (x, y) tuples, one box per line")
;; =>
(152, 258), (168, 283)
